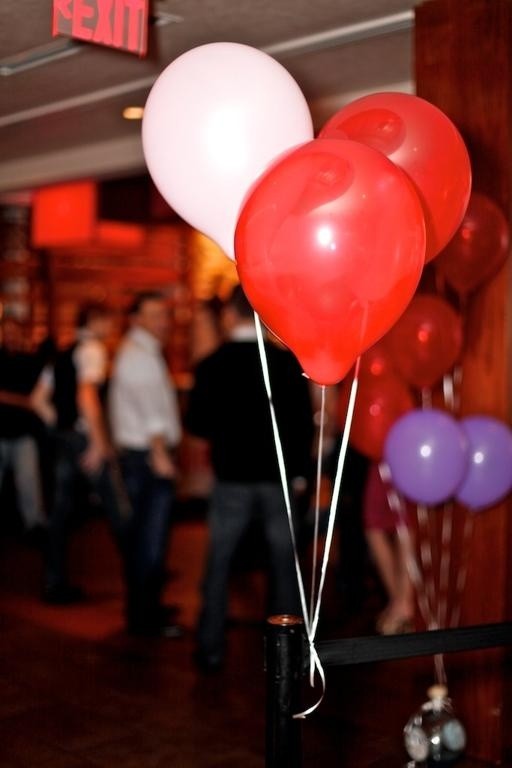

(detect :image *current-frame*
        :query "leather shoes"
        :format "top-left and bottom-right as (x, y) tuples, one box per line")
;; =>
(163, 623), (184, 640)
(191, 645), (224, 676)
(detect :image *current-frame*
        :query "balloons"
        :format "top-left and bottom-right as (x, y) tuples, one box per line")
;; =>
(452, 415), (512, 516)
(436, 189), (510, 296)
(385, 409), (471, 507)
(384, 292), (462, 390)
(234, 136), (427, 385)
(316, 91), (472, 262)
(140, 41), (314, 263)
